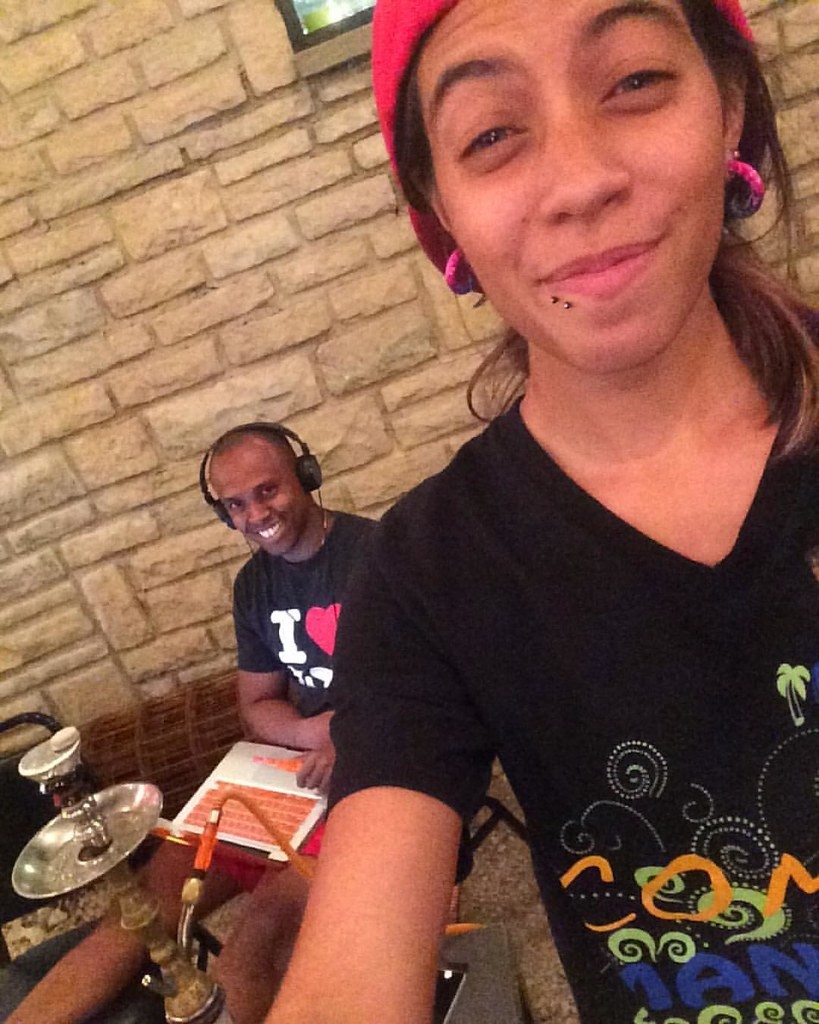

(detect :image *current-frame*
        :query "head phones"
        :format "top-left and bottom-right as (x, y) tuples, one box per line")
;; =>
(199, 422), (323, 530)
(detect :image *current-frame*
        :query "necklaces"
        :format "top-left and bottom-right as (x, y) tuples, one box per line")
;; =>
(321, 510), (328, 545)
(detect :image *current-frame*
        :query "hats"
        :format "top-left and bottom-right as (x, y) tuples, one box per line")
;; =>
(371, 0), (752, 300)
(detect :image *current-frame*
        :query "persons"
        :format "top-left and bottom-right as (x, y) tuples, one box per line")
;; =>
(0, 422), (384, 1024)
(262, 1), (819, 1024)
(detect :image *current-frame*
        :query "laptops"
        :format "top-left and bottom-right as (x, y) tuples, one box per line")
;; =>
(146, 740), (330, 863)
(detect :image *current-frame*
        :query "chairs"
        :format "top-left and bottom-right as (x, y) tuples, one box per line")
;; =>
(0, 713), (223, 1024)
